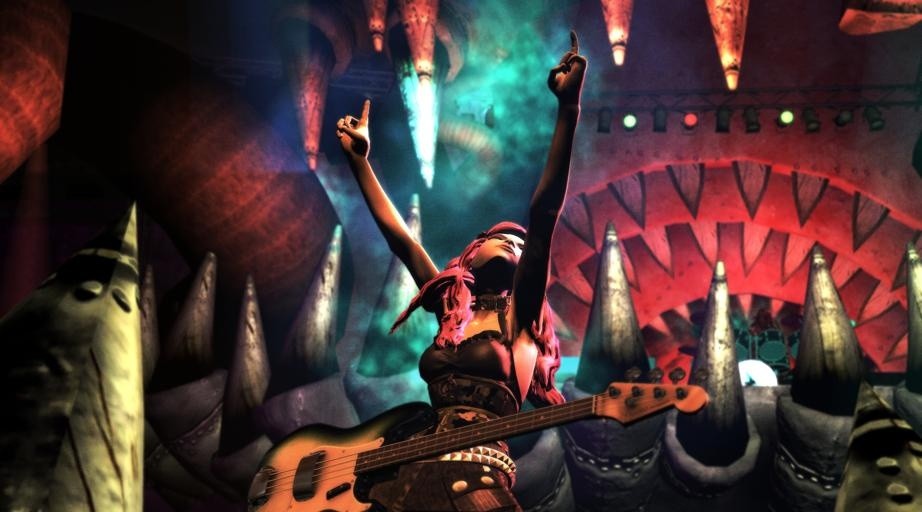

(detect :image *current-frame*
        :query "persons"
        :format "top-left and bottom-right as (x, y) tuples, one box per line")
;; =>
(335, 30), (587, 512)
